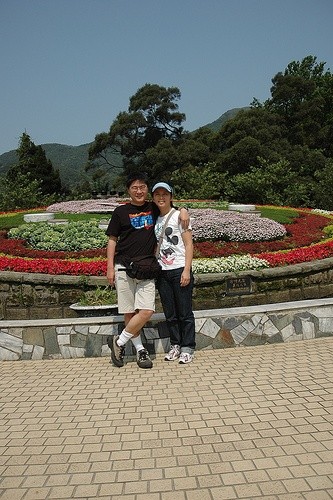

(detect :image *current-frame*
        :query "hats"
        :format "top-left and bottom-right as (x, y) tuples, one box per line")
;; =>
(151, 182), (172, 193)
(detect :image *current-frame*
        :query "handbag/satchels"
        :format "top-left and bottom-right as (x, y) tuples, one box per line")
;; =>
(123, 256), (162, 280)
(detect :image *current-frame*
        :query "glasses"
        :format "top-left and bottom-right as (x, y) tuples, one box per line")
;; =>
(130, 186), (147, 190)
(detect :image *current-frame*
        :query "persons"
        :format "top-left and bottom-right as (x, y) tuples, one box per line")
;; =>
(150, 182), (195, 364)
(104, 171), (189, 369)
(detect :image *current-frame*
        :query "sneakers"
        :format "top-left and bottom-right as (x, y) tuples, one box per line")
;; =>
(106, 335), (126, 367)
(164, 345), (181, 361)
(136, 349), (153, 369)
(178, 351), (194, 364)
